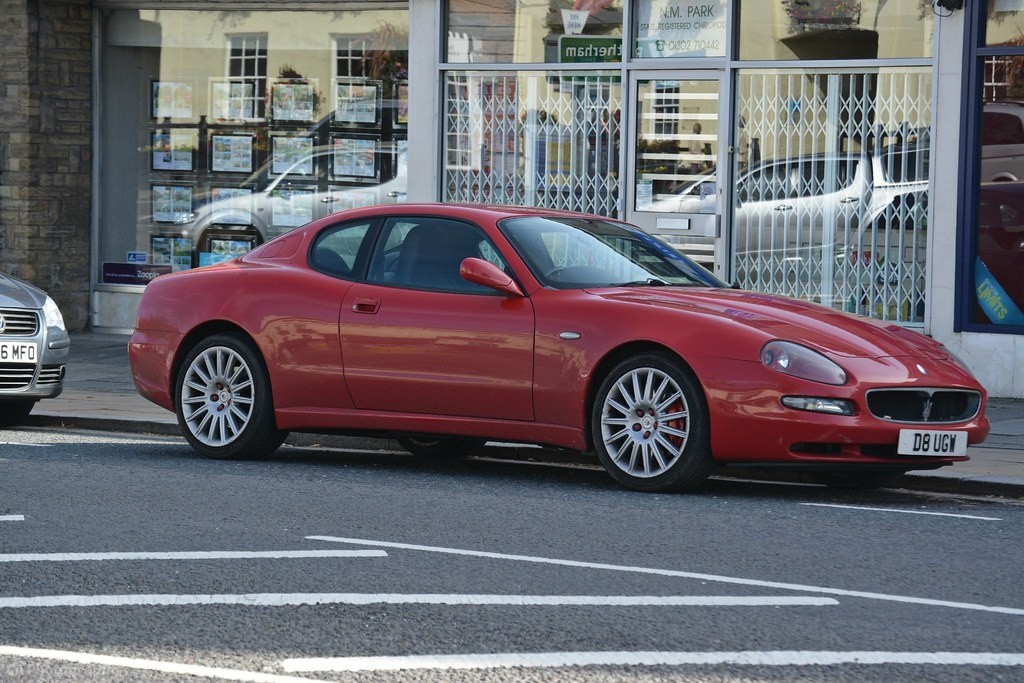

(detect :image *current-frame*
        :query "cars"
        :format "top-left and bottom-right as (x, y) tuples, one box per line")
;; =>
(0, 271), (71, 422)
(138, 99), (422, 269)
(638, 101), (1024, 318)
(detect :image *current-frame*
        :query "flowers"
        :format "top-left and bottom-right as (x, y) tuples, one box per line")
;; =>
(781, 0), (862, 34)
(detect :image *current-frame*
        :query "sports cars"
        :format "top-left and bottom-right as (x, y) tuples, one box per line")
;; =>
(128, 203), (991, 492)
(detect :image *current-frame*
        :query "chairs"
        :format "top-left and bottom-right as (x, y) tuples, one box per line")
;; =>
(396, 224), (467, 292)
